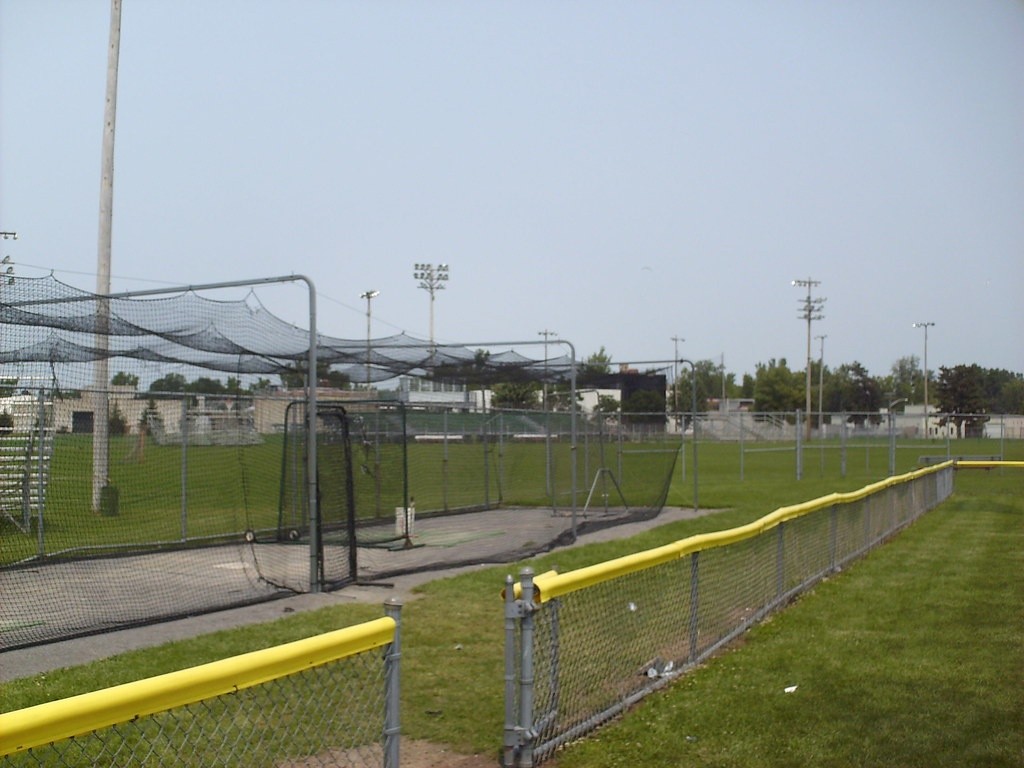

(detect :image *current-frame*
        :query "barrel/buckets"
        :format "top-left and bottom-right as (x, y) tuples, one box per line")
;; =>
(395, 507), (415, 536)
(101, 487), (119, 515)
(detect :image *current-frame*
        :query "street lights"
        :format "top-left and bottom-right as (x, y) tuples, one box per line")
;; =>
(361, 291), (381, 391)
(814, 335), (828, 438)
(913, 322), (936, 439)
(792, 276), (826, 441)
(887, 398), (908, 438)
(671, 336), (686, 412)
(538, 330), (557, 410)
(413, 264), (448, 391)
